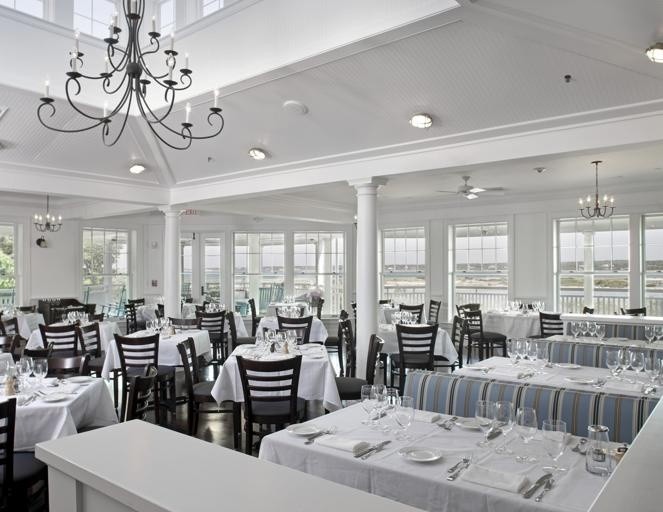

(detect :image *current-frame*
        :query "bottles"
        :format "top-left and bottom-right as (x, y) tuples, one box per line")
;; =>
(583, 423), (612, 477)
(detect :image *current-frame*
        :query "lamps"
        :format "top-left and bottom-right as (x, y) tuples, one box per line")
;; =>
(578, 162), (615, 219)
(33, 196), (63, 232)
(38, 1), (224, 149)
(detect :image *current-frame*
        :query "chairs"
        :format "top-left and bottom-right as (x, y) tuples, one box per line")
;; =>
(124, 363), (157, 424)
(337, 334), (384, 406)
(177, 337), (244, 443)
(237, 354), (309, 457)
(116, 330), (176, 422)
(0, 397), (49, 512)
(1, 282), (663, 395)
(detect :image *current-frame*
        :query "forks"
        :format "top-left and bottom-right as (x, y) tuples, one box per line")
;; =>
(438, 413), (456, 428)
(445, 453), (471, 485)
(307, 426), (336, 441)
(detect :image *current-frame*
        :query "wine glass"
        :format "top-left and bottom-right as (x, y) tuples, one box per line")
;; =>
(604, 349), (662, 394)
(496, 300), (544, 313)
(145, 316), (170, 337)
(7, 357), (53, 399)
(206, 295), (302, 317)
(642, 324), (662, 347)
(356, 380), (411, 443)
(503, 335), (537, 372)
(390, 310), (416, 327)
(569, 319), (605, 343)
(473, 399), (567, 474)
(260, 327), (299, 356)
(1, 303), (89, 327)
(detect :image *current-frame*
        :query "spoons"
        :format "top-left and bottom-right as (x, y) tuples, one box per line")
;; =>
(571, 435), (585, 454)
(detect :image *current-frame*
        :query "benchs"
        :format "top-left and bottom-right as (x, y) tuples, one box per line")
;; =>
(402, 367), (658, 444)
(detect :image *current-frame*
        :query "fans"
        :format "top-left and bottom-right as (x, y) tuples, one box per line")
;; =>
(435, 176), (504, 200)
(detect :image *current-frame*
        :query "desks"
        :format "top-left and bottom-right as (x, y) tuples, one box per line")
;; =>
(6, 373), (111, 479)
(35, 418), (424, 511)
(261, 397), (632, 512)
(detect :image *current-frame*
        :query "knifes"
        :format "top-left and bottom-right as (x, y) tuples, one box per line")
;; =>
(523, 471), (552, 501)
(353, 439), (392, 458)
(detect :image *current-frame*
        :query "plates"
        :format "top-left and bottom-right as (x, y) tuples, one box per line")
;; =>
(562, 375), (593, 386)
(286, 424), (319, 435)
(455, 416), (480, 430)
(555, 362), (581, 370)
(396, 446), (441, 462)
(43, 375), (90, 404)
